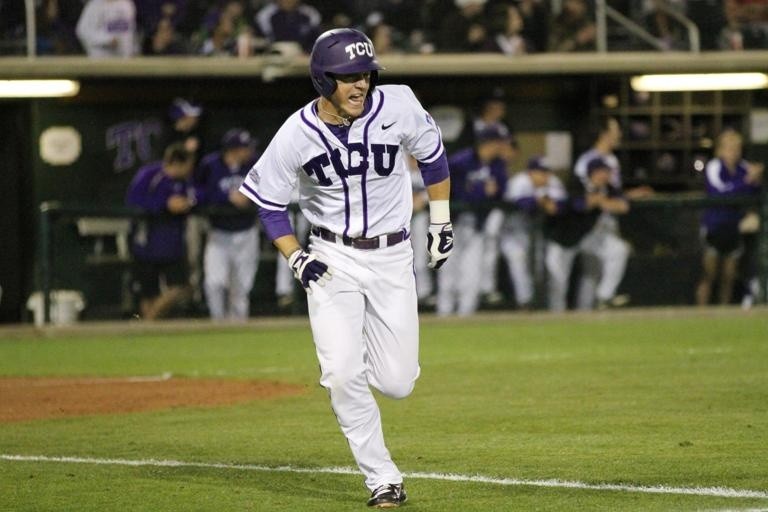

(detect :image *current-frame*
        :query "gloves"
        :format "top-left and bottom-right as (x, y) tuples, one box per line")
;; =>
(425, 222), (455, 269)
(287, 250), (331, 294)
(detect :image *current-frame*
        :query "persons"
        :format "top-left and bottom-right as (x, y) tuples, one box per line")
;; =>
(694, 126), (763, 303)
(125, 96), (262, 325)
(75, 0), (256, 57)
(236, 24), (455, 509)
(260, 2), (409, 56)
(411, 87), (656, 312)
(411, 1), (596, 55)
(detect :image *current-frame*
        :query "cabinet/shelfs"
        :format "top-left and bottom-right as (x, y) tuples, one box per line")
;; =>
(558, 78), (767, 303)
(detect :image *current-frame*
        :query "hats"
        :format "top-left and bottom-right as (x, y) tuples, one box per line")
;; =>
(219, 129), (258, 147)
(527, 155), (552, 170)
(167, 101), (202, 119)
(470, 120), (509, 144)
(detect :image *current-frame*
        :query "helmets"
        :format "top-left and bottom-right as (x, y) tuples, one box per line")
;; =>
(309, 28), (387, 97)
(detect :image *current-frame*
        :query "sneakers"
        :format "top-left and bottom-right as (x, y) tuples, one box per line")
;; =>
(366, 483), (407, 508)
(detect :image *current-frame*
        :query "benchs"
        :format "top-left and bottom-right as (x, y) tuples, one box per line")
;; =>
(74, 212), (286, 312)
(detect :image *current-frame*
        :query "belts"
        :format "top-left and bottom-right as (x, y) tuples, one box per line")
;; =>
(313, 226), (406, 250)
